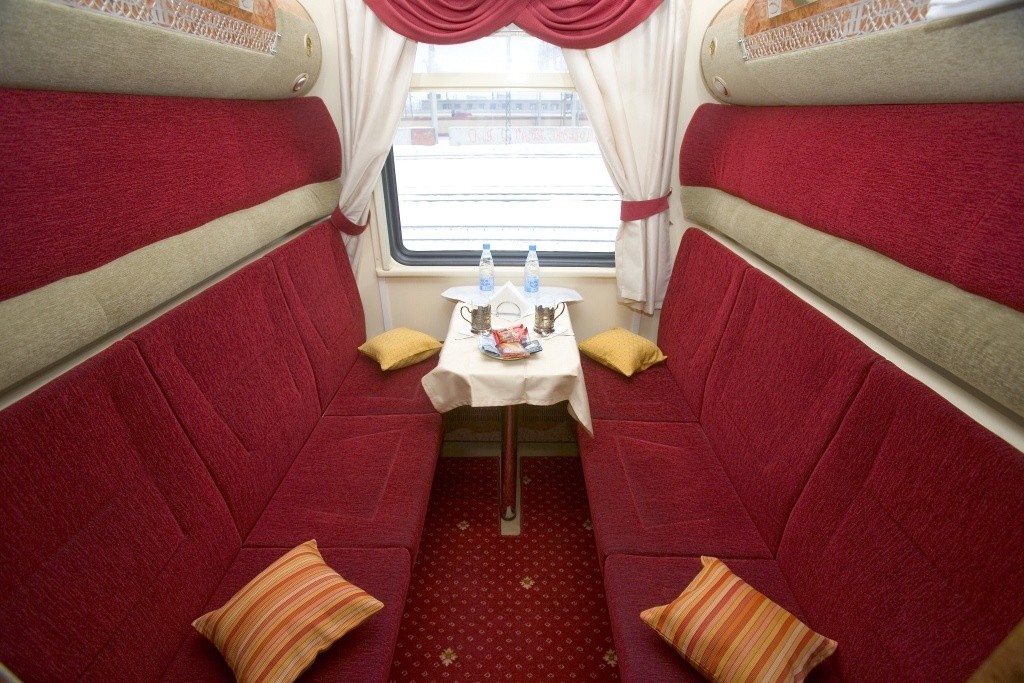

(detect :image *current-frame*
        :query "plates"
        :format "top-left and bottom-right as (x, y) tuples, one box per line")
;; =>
(479, 345), (525, 361)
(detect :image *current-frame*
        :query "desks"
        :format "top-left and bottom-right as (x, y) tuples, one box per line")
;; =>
(421, 289), (593, 535)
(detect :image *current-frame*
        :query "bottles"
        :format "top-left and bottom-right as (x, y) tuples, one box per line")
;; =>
(522, 244), (541, 300)
(478, 243), (495, 298)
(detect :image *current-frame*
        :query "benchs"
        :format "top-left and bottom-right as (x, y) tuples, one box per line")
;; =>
(568, 95), (1024, 682)
(1, 96), (449, 683)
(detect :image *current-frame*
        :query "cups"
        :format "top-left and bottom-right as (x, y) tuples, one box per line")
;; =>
(533, 295), (565, 334)
(460, 297), (492, 334)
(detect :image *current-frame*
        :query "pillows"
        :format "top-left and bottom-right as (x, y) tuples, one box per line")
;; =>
(358, 327), (442, 371)
(639, 556), (838, 682)
(578, 327), (668, 376)
(190, 538), (385, 682)
